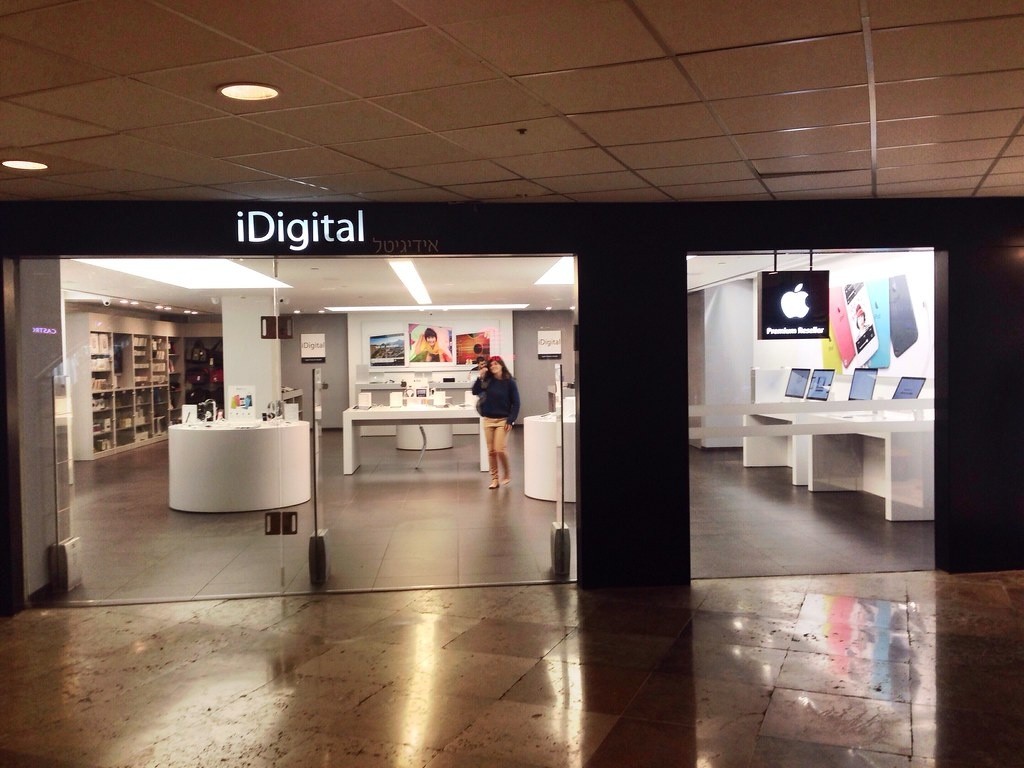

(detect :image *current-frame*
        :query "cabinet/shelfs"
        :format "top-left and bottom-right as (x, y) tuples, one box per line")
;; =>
(73, 317), (185, 461)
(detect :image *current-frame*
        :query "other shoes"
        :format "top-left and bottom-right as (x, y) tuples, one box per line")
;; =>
(489, 479), (499, 489)
(502, 476), (510, 485)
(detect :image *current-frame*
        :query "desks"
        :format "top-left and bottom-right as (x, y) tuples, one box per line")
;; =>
(166, 422), (309, 512)
(739, 411), (934, 522)
(523, 413), (576, 502)
(342, 404), (489, 475)
(395, 425), (453, 451)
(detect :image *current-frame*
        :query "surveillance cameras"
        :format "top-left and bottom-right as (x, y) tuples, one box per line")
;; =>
(102, 300), (112, 306)
(279, 297), (285, 303)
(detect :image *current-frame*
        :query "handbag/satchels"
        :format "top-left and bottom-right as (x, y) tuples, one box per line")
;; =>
(185, 337), (224, 409)
(476, 378), (489, 417)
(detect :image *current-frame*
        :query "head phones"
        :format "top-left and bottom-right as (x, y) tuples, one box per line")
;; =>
(268, 400), (282, 419)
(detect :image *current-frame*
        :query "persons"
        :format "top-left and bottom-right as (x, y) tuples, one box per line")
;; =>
(415, 327), (445, 362)
(471, 356), (520, 489)
(856, 304), (868, 336)
(472, 356), (488, 371)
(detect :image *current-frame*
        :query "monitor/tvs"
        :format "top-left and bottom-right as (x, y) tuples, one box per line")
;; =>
(369, 324), (491, 368)
(783, 368), (926, 401)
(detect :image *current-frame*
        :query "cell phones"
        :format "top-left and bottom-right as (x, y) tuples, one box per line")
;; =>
(821, 275), (918, 374)
(231, 394), (252, 409)
